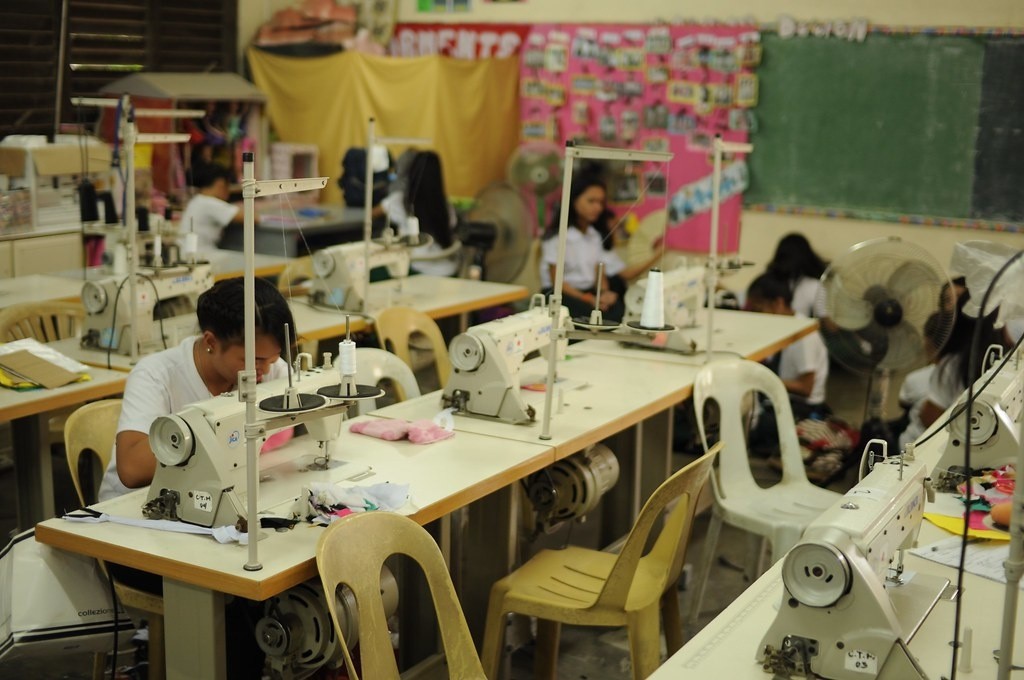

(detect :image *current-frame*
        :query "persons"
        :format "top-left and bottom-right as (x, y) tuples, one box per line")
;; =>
(746, 270), (836, 461)
(95, 274), (299, 680)
(175, 161), (262, 266)
(854, 272), (1016, 478)
(742, 230), (843, 337)
(370, 148), (463, 279)
(535, 171), (669, 346)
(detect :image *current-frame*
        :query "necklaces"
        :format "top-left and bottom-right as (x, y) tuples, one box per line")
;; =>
(196, 335), (235, 399)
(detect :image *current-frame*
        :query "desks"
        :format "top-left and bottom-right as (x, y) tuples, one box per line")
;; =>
(0, 231), (1024, 678)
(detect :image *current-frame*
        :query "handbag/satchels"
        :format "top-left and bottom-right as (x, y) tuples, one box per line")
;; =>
(0, 523), (139, 665)
